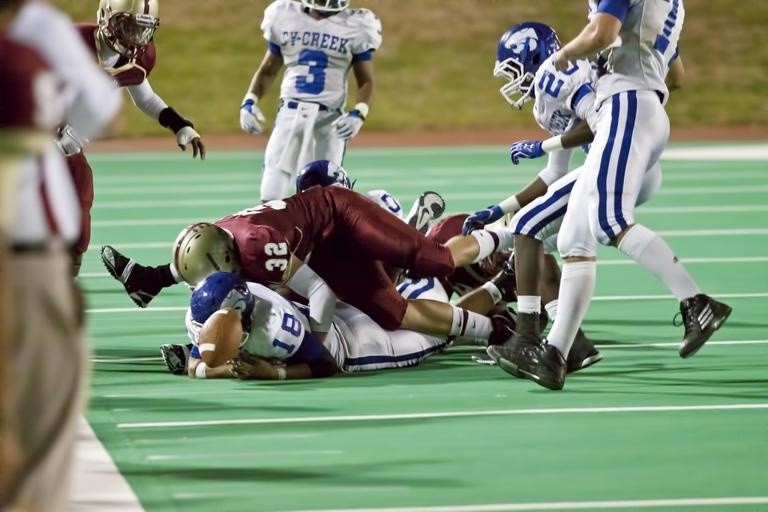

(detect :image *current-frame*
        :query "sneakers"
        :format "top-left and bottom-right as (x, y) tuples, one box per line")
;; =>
(465, 245), (732, 390)
(161, 344), (192, 374)
(102, 244), (158, 308)
(405, 192), (445, 233)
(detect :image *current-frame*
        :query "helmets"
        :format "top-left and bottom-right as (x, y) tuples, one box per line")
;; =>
(190, 272), (253, 352)
(302, 0), (349, 13)
(492, 22), (561, 109)
(296, 159), (351, 192)
(96, 0), (158, 59)
(172, 221), (243, 289)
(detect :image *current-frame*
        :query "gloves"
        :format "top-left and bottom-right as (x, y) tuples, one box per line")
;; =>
(239, 97), (265, 133)
(332, 110), (365, 140)
(510, 140), (542, 165)
(461, 204), (503, 235)
(57, 125), (89, 156)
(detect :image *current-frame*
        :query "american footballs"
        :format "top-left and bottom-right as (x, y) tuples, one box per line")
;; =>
(199, 307), (243, 368)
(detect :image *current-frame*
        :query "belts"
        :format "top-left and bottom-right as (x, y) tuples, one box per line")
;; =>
(287, 101), (325, 111)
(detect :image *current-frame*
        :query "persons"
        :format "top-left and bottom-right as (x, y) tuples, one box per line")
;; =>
(47, 1), (203, 281)
(239, 0), (384, 205)
(1, 0), (144, 512)
(459, 22), (661, 377)
(487, 1), (733, 391)
(98, 157), (574, 379)
